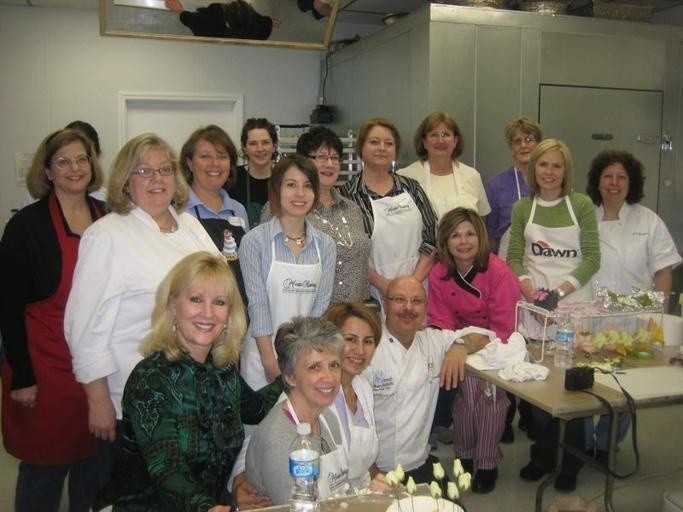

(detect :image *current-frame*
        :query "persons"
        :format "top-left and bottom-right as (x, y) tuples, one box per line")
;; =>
(484, 114), (544, 444)
(174, 122), (254, 330)
(233, 114), (278, 232)
(361, 277), (497, 512)
(63, 131), (271, 511)
(239, 314), (403, 505)
(109, 248), (289, 512)
(336, 114), (441, 324)
(0, 127), (109, 508)
(501, 137), (601, 497)
(582, 149), (682, 470)
(223, 150), (339, 496)
(259, 125), (372, 312)
(63, 121), (109, 209)
(224, 297), (381, 511)
(394, 108), (491, 454)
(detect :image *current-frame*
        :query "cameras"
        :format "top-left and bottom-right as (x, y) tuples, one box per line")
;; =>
(564, 366), (595, 390)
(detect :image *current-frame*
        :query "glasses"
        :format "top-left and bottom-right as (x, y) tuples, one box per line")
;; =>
(306, 152), (342, 163)
(512, 136), (538, 145)
(384, 295), (426, 304)
(49, 153), (96, 171)
(130, 166), (176, 179)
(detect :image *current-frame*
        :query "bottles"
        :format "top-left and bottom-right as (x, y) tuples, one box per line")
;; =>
(553, 313), (576, 367)
(287, 423), (320, 509)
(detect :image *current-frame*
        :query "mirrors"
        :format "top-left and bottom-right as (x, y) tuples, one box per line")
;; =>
(98, 0), (341, 52)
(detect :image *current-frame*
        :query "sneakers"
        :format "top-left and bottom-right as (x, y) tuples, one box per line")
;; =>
(555, 454), (583, 494)
(519, 444), (554, 483)
(585, 448), (610, 467)
(453, 455), (498, 494)
(427, 424), (456, 452)
(499, 416), (532, 445)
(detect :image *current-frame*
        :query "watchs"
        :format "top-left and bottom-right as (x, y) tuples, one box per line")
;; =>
(453, 338), (467, 352)
(556, 287), (565, 299)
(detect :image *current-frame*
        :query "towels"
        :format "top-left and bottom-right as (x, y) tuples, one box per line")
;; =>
(461, 329), (529, 374)
(496, 359), (550, 386)
(590, 359), (683, 403)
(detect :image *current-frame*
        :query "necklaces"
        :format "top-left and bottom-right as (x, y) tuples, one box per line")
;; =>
(282, 232), (305, 246)
(157, 222), (175, 233)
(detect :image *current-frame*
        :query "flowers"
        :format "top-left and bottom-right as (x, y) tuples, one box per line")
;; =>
(381, 457), (474, 512)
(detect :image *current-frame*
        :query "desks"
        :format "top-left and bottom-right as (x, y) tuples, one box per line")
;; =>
(450, 334), (682, 511)
(249, 474), (462, 512)
